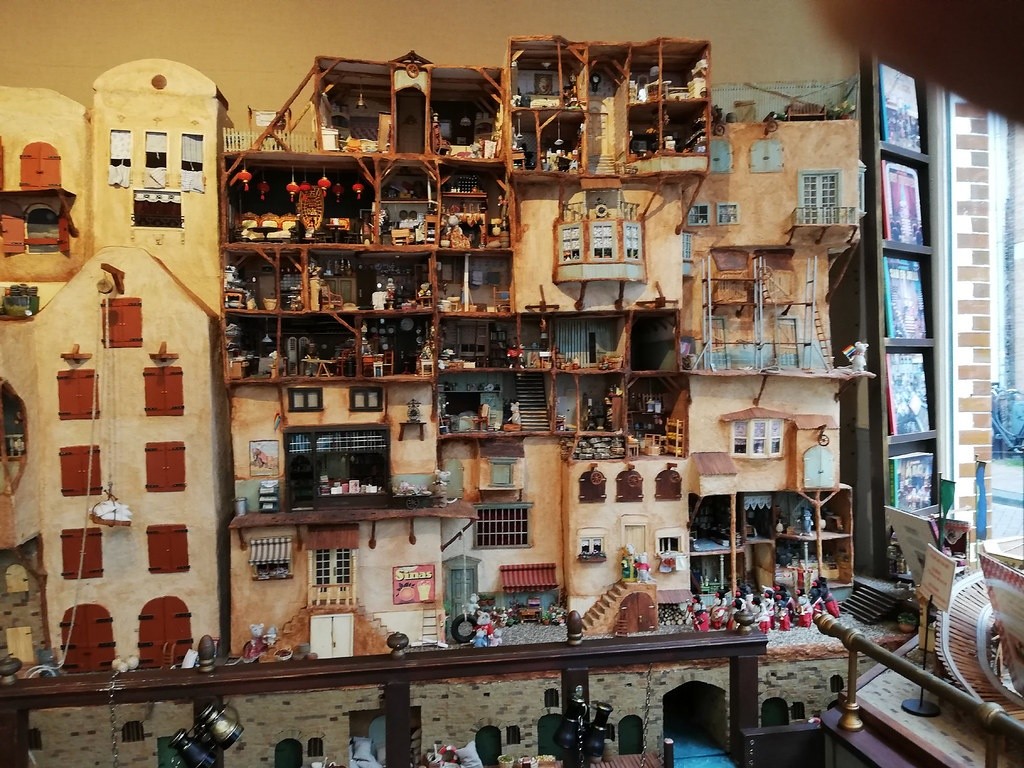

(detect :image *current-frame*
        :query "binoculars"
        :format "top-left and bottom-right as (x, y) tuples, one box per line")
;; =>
(555, 698), (614, 761)
(170, 701), (246, 768)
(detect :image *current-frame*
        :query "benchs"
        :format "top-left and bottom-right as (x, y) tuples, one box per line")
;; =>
(312, 233), (325, 243)
(348, 233), (363, 244)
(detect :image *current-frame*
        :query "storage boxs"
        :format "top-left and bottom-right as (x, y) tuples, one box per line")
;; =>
(438, 384), (445, 392)
(330, 487), (342, 495)
(3, 295), (40, 317)
(647, 446), (660, 457)
(366, 486), (377, 493)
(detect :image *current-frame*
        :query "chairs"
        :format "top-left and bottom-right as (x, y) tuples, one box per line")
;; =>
(383, 351), (394, 376)
(472, 402), (492, 430)
(337, 349), (352, 376)
(234, 212), (298, 242)
(321, 288), (343, 311)
(436, 127), (451, 155)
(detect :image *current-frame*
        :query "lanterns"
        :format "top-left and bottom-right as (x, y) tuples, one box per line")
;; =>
(238, 170), (363, 203)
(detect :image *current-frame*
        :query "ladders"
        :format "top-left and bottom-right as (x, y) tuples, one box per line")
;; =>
(756, 272), (772, 302)
(814, 306), (832, 369)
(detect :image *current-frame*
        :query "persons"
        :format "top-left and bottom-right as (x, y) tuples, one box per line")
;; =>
(685, 581), (836, 632)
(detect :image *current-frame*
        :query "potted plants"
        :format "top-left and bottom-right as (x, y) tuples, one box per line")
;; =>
(542, 612), (551, 624)
(497, 754), (514, 768)
(606, 351), (623, 369)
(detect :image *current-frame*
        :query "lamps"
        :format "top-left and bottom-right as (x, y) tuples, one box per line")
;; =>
(460, 115), (472, 126)
(516, 116), (524, 140)
(261, 317), (272, 343)
(554, 117), (564, 145)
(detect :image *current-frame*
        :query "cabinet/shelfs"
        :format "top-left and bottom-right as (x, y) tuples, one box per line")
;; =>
(226, 335), (248, 380)
(213, 34), (855, 664)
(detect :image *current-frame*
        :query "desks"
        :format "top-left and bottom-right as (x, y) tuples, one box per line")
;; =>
(326, 223), (345, 243)
(363, 355), (382, 376)
(519, 608), (542, 623)
(628, 444), (639, 456)
(424, 214), (437, 245)
(224, 292), (245, 308)
(247, 226), (283, 243)
(420, 358), (434, 378)
(301, 359), (336, 378)
(740, 722), (825, 768)
(669, 87), (689, 92)
(512, 150), (526, 170)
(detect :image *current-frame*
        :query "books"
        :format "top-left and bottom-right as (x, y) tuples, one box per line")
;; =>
(880, 64), (935, 509)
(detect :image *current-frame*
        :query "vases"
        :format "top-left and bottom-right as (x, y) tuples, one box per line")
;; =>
(307, 652), (317, 660)
(262, 299), (278, 310)
(298, 643), (312, 653)
(579, 556), (606, 563)
(291, 653), (306, 661)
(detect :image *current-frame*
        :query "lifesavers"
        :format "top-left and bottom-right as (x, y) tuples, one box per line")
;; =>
(594, 204), (607, 218)
(451, 613), (476, 641)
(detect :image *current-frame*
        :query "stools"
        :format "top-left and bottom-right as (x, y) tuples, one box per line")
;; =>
(390, 229), (409, 245)
(373, 361), (383, 377)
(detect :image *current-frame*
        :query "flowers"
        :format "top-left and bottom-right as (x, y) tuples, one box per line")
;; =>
(578, 550), (607, 560)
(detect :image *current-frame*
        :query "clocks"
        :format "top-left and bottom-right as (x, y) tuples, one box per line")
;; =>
(590, 72), (601, 92)
